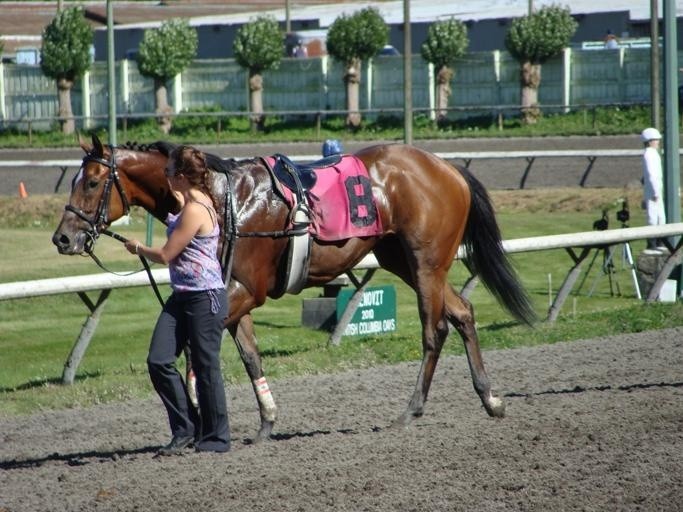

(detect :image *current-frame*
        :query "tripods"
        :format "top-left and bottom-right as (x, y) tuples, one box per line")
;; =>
(573, 228), (619, 298)
(587, 223), (646, 300)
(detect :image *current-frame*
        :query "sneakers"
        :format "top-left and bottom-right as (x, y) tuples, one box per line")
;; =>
(159, 436), (196, 453)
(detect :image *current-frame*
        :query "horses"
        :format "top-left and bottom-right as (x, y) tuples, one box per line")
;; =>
(52, 133), (539, 445)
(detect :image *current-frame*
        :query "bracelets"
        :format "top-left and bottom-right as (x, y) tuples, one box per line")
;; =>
(135, 243), (142, 254)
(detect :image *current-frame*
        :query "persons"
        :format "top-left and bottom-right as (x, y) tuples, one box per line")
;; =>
(292, 39), (308, 58)
(323, 139), (348, 332)
(640, 128), (666, 225)
(605, 34), (618, 48)
(124, 144), (231, 455)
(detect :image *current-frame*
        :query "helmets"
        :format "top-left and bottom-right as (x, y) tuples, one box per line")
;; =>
(641, 128), (662, 143)
(323, 140), (344, 157)
